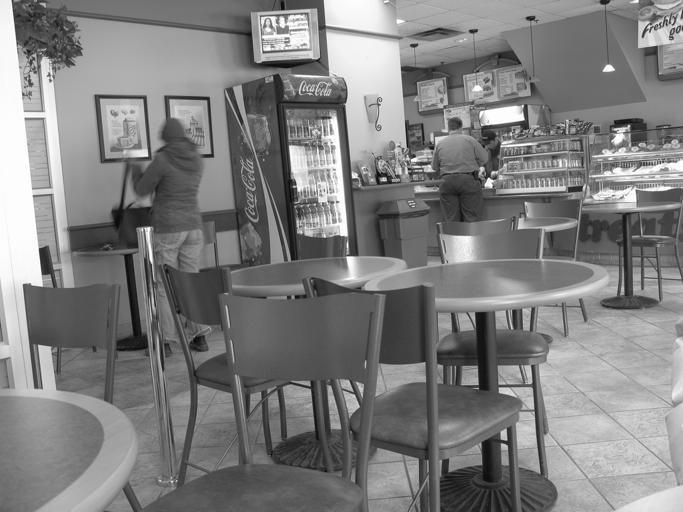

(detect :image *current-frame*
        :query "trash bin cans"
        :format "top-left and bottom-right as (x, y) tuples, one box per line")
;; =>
(377, 197), (431, 268)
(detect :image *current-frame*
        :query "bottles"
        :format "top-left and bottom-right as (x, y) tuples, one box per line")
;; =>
(286, 109), (343, 238)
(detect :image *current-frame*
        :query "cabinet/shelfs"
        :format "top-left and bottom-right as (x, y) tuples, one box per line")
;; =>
(495, 125), (683, 190)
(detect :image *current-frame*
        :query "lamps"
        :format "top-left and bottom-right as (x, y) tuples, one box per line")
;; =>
(468, 28), (483, 92)
(526, 16), (541, 83)
(599, 0), (616, 74)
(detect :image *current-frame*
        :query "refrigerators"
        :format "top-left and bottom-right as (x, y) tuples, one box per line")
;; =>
(223, 72), (358, 270)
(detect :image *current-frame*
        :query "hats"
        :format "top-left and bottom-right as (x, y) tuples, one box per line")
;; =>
(162, 119), (185, 141)
(482, 130), (496, 145)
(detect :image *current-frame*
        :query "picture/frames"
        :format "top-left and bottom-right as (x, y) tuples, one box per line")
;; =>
(409, 123), (424, 147)
(164, 95), (215, 160)
(94, 94), (152, 164)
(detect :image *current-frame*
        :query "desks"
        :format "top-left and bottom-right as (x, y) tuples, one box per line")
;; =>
(1, 387), (140, 511)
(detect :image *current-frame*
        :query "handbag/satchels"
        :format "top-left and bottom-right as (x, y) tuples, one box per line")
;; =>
(112, 206), (151, 248)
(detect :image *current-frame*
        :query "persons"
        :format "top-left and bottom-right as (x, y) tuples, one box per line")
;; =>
(479, 129), (504, 179)
(431, 116), (489, 223)
(126, 117), (212, 359)
(277, 15), (289, 35)
(262, 16), (275, 35)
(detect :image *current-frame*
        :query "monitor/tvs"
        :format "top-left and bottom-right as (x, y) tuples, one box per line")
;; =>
(250, 7), (321, 67)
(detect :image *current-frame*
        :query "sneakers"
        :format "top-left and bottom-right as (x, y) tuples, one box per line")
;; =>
(145, 344), (175, 357)
(190, 334), (209, 352)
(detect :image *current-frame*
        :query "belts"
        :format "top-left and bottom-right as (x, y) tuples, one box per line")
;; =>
(443, 172), (473, 176)
